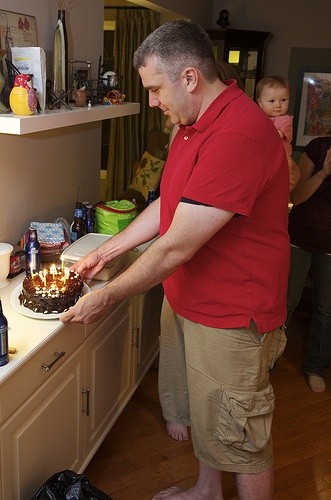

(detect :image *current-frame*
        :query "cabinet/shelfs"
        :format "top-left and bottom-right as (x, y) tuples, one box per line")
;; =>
(205, 28), (273, 104)
(1, 225), (165, 500)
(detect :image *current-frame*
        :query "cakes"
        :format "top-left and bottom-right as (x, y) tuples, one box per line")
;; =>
(18, 268), (84, 314)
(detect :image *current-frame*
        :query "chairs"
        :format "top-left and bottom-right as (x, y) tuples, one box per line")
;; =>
(95, 55), (124, 103)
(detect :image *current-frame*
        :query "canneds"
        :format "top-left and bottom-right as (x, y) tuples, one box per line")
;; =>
(9, 245), (22, 275)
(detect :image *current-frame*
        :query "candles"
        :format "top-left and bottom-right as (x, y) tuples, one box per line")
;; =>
(28, 252), (70, 286)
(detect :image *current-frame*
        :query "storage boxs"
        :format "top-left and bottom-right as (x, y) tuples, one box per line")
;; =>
(59, 233), (131, 282)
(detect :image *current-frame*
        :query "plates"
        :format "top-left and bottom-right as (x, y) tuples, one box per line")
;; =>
(11, 282), (91, 319)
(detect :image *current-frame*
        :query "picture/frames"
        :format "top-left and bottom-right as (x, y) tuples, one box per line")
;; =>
(284, 45), (331, 153)
(67, 59), (92, 104)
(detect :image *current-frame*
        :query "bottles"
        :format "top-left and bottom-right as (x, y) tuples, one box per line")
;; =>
(25, 228), (39, 276)
(83, 205), (95, 235)
(70, 202), (82, 232)
(82, 201), (90, 216)
(0, 299), (9, 367)
(70, 211), (86, 244)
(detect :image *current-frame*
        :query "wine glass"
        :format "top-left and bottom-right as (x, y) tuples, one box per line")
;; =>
(0, 243), (13, 288)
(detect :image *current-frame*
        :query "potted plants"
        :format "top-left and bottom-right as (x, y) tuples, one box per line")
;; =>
(72, 74), (88, 107)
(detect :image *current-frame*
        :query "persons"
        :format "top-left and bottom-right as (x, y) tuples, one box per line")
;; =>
(157, 62), (301, 442)
(58, 18), (288, 500)
(269, 126), (331, 393)
(255, 74), (295, 214)
